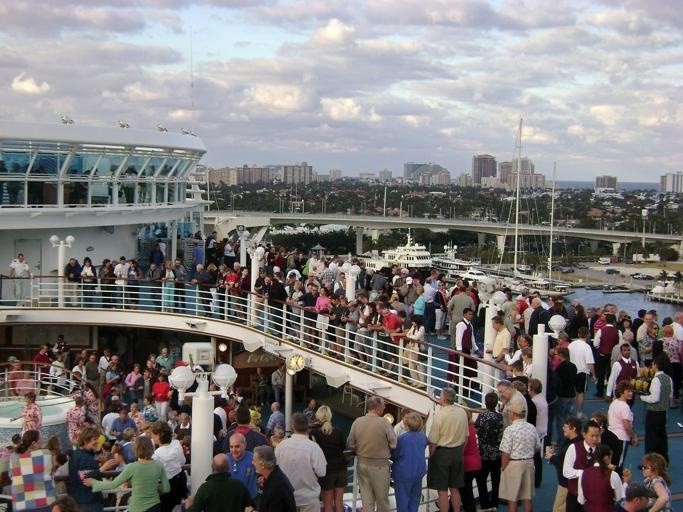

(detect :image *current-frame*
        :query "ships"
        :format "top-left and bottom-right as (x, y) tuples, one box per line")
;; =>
(357, 227), (432, 278)
(432, 240), (487, 282)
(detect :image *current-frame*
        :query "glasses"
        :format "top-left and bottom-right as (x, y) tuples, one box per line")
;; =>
(499, 388), (510, 395)
(642, 464), (656, 470)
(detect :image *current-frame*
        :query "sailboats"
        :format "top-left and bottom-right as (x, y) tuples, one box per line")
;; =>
(482, 115), (575, 300)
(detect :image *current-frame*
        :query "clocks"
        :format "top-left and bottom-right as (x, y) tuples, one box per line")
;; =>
(285, 352), (306, 372)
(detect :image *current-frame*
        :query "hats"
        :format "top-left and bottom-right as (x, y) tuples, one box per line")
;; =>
(625, 481), (658, 502)
(506, 400), (526, 415)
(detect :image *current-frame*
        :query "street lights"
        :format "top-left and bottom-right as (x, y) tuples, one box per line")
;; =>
(532, 315), (567, 399)
(49, 234), (75, 307)
(238, 230), (250, 267)
(168, 363), (238, 499)
(341, 262), (361, 302)
(247, 246), (265, 325)
(478, 290), (505, 408)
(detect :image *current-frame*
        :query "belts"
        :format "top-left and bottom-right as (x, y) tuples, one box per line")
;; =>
(507, 457), (534, 461)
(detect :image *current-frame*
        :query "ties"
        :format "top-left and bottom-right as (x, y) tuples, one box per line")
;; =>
(588, 447), (594, 459)
(627, 360), (630, 365)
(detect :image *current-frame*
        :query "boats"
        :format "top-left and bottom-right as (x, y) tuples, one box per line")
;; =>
(601, 284), (633, 293)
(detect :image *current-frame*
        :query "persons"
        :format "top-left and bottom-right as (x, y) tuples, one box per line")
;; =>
(172, 258), (188, 315)
(290, 280), (304, 344)
(350, 259), (363, 288)
(274, 272), (284, 286)
(485, 316), (511, 400)
(671, 311), (683, 341)
(161, 260), (173, 312)
(369, 304), (401, 378)
(230, 393), (242, 406)
(92, 350), (100, 363)
(0, 156), (188, 210)
(245, 446), (297, 512)
(308, 266), (322, 277)
(218, 264), (228, 321)
(64, 396), (86, 451)
(546, 359), (559, 444)
(303, 400), (320, 432)
(135, 369), (154, 409)
(605, 381), (638, 475)
(547, 295), (556, 310)
(636, 357), (674, 470)
(15, 369), (36, 395)
(527, 378), (547, 484)
(267, 279), (292, 338)
(224, 434), (258, 500)
(187, 232), (193, 239)
(172, 430), (182, 439)
(578, 444), (632, 512)
(180, 397), (193, 413)
(96, 442), (112, 472)
(287, 246), (308, 276)
(204, 229), (241, 264)
(48, 433), (62, 454)
(265, 402), (286, 436)
(567, 305), (589, 339)
(119, 427), (138, 463)
(502, 288), (513, 301)
(504, 333), (533, 367)
(149, 244), (163, 265)
(316, 293), (336, 357)
(271, 364), (285, 403)
(333, 296), (349, 360)
(232, 262), (241, 278)
(533, 290), (547, 309)
(296, 285), (319, 348)
(128, 260), (142, 310)
(335, 279), (347, 296)
(502, 303), (517, 357)
(223, 399), (236, 411)
(340, 301), (359, 362)
(52, 334), (70, 369)
(555, 296), (565, 304)
(112, 453), (129, 472)
(447, 286), (475, 350)
(471, 281), (484, 344)
(610, 330), (638, 372)
(329, 256), (340, 272)
(358, 301), (378, 372)
(142, 397), (159, 423)
(235, 266), (251, 324)
(523, 298), (534, 335)
(73, 382), (100, 418)
(254, 269), (267, 329)
(273, 413), (327, 512)
(393, 412), (429, 511)
(412, 271), (423, 287)
(658, 326), (682, 408)
(450, 279), (464, 297)
(394, 269), (409, 291)
(50, 356), (67, 396)
(618, 316), (638, 350)
(521, 348), (534, 376)
(406, 315), (426, 390)
(114, 256), (130, 310)
(102, 403), (120, 444)
(85, 355), (99, 391)
(504, 361), (529, 382)
(310, 405), (350, 512)
(146, 264), (161, 312)
(589, 412), (623, 470)
(246, 399), (263, 433)
(378, 265), (397, 282)
(651, 340), (671, 378)
(548, 421), (583, 511)
(182, 453), (251, 512)
(658, 317), (676, 336)
(156, 347), (173, 374)
(191, 264), (214, 318)
(51, 454), (67, 474)
(106, 355), (126, 380)
(142, 360), (159, 383)
(390, 310), (413, 384)
(191, 240), (204, 264)
(515, 292), (529, 315)
(649, 310), (658, 321)
(65, 257), (81, 306)
(428, 388), (470, 512)
(612, 305), (617, 316)
(124, 364), (143, 391)
(510, 300), (525, 350)
(152, 367), (169, 383)
(109, 260), (117, 309)
(329, 295), (340, 319)
(433, 282), (448, 340)
(111, 443), (121, 455)
(593, 310), (608, 334)
(454, 307), (483, 396)
(73, 370), (87, 383)
(424, 277), (437, 335)
(152, 421), (188, 506)
(631, 309), (647, 362)
(637, 313), (655, 354)
(498, 378), (528, 425)
(103, 386), (128, 410)
(7, 429), (59, 512)
(213, 404), (268, 454)
(74, 349), (87, 367)
(605, 343), (646, 410)
(553, 348), (577, 443)
(224, 268), (245, 323)
(102, 396), (120, 418)
(639, 323), (657, 367)
(614, 483), (653, 512)
(462, 408), (481, 512)
(98, 259), (111, 309)
(166, 411), (177, 431)
(552, 303), (565, 318)
(393, 268), (400, 284)
(378, 295), (387, 304)
(8, 433), (22, 447)
(306, 282), (314, 293)
(81, 436), (170, 512)
(152, 374), (172, 423)
(590, 306), (601, 337)
(303, 272), (320, 293)
(564, 423), (617, 512)
(304, 287), (331, 352)
(572, 299), (581, 309)
(128, 402), (144, 431)
(401, 277), (417, 321)
(210, 384), (219, 403)
(194, 233), (201, 240)
(263, 275), (271, 298)
(408, 286), (425, 325)
(137, 423), (155, 448)
(472, 393), (504, 512)
(73, 357), (87, 381)
(1, 445), (14, 458)
(455, 280), (479, 307)
(498, 399), (541, 511)
(424, 269), (440, 289)
(9, 392), (42, 434)
(197, 231), (203, 240)
(547, 335), (560, 370)
(370, 269), (390, 300)
(593, 314), (622, 398)
(502, 294), (512, 323)
(586, 307), (593, 330)
(347, 397), (396, 512)
(239, 398), (253, 409)
(109, 407), (138, 442)
(96, 348), (114, 394)
(369, 303), (386, 375)
(102, 361), (120, 397)
(559, 332), (569, 350)
(603, 304), (613, 316)
(516, 288), (529, 304)
(334, 273), (346, 292)
(149, 354), (162, 372)
(258, 242), (286, 273)
(354, 293), (371, 369)
(8, 360), (24, 396)
(226, 410), (240, 431)
(309, 255), (316, 275)
(9, 253), (32, 305)
(364, 266), (374, 292)
(158, 239), (166, 256)
(315, 256), (325, 274)
(434, 273), (446, 288)
(102, 379), (120, 397)
(81, 256), (98, 309)
(388, 263), (398, 295)
(510, 381), (537, 426)
(213, 398), (227, 439)
(207, 264), (219, 311)
(288, 272), (305, 303)
(529, 299), (554, 346)
(618, 311), (626, 324)
(357, 258), (369, 291)
(639, 452), (673, 512)
(173, 413), (191, 437)
(55, 448), (74, 476)
(73, 416), (94, 449)
(66, 427), (118, 512)
(567, 327), (596, 420)
(250, 366), (271, 407)
(175, 232), (185, 258)
(178, 436), (190, 463)
(393, 407), (413, 436)
(32, 345), (53, 396)
